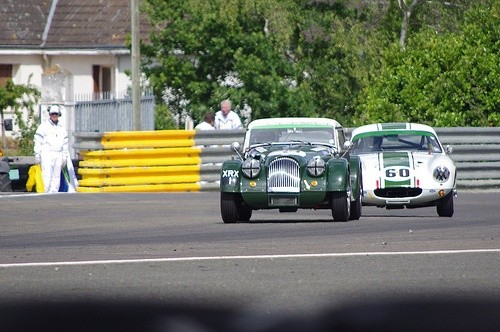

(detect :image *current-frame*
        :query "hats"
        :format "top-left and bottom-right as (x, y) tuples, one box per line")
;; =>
(50, 106), (59, 113)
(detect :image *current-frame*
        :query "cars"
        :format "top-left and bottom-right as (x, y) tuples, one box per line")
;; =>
(219, 117), (364, 223)
(343, 122), (458, 221)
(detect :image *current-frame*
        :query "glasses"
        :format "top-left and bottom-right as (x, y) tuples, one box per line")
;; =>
(51, 113), (59, 116)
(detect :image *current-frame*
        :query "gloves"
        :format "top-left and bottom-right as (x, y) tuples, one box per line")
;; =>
(35, 153), (41, 165)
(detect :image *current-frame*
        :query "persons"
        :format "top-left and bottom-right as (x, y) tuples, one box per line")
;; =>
(33, 105), (69, 193)
(194, 111), (218, 148)
(259, 130), (276, 143)
(367, 135), (384, 151)
(213, 98), (243, 168)
